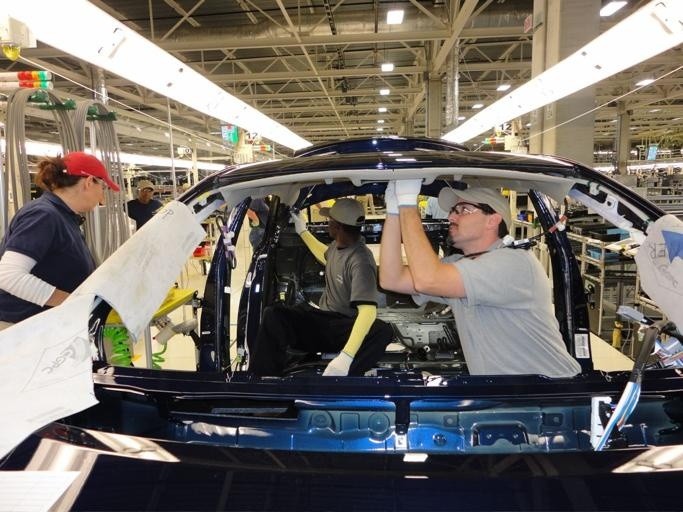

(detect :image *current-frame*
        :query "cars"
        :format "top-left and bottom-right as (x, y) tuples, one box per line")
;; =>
(0, 138), (683, 512)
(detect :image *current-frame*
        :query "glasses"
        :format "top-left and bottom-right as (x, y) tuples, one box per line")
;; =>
(82, 168), (108, 191)
(448, 202), (483, 215)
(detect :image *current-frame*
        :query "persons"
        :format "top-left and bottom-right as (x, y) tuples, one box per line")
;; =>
(248, 198), (270, 252)
(247, 198), (396, 381)
(123, 179), (163, 230)
(0, 152), (120, 332)
(378, 180), (584, 379)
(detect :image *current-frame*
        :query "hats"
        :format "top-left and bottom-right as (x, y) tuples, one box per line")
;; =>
(137, 180), (156, 191)
(438, 187), (512, 231)
(62, 151), (120, 193)
(319, 198), (365, 227)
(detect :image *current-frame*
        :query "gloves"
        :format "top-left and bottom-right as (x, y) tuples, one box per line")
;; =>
(385, 177), (423, 215)
(321, 351), (353, 376)
(288, 209), (309, 235)
(250, 221), (260, 226)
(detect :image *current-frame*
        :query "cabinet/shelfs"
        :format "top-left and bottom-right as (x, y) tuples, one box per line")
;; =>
(513, 219), (668, 364)
